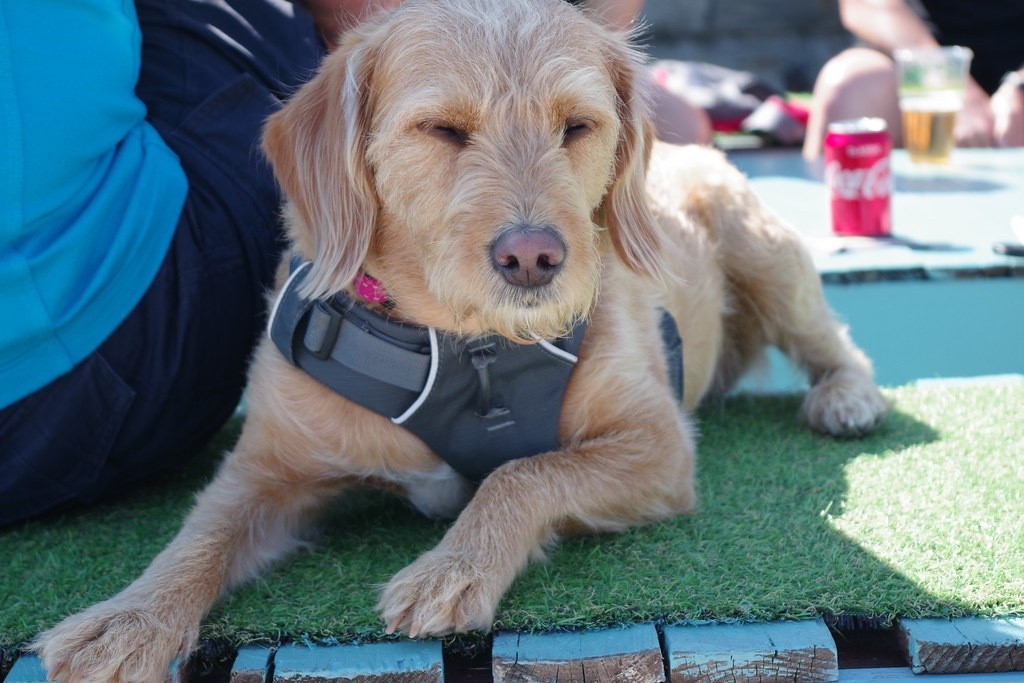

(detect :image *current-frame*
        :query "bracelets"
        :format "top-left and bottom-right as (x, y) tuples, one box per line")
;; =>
(1000, 71), (1024, 89)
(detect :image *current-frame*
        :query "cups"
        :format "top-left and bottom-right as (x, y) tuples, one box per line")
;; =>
(896, 46), (974, 164)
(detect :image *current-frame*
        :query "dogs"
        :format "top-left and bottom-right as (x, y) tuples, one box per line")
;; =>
(34, 1), (894, 683)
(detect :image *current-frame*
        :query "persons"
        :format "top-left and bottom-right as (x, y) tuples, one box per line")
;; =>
(0, 0), (329, 535)
(803, 0), (1024, 161)
(311, 0), (713, 145)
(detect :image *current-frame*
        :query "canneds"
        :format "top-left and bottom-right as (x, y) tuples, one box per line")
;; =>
(826, 115), (892, 236)
